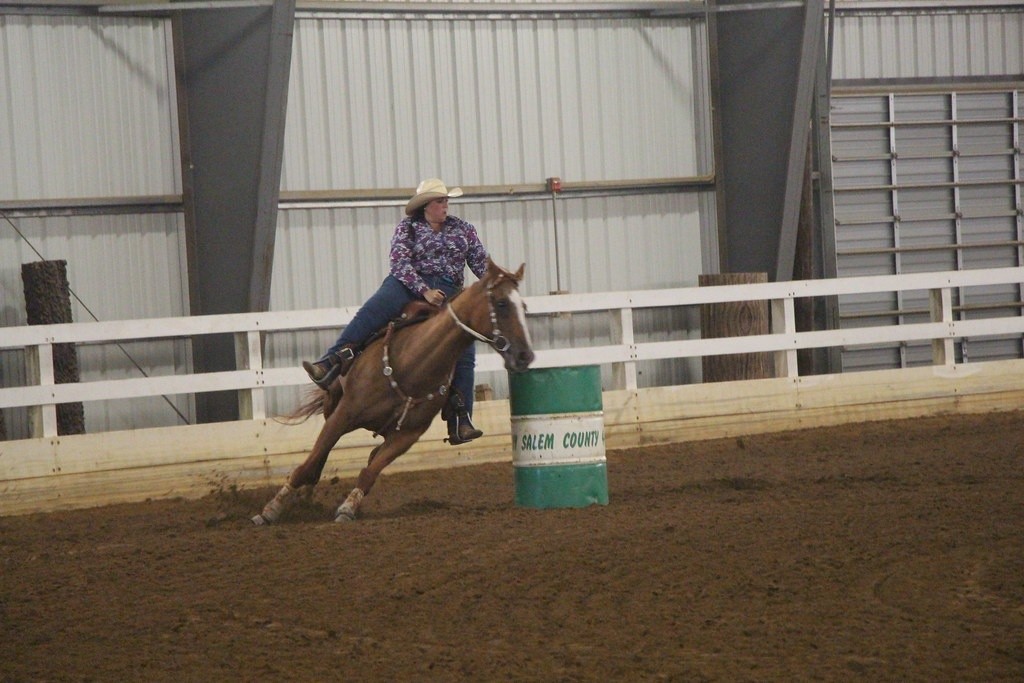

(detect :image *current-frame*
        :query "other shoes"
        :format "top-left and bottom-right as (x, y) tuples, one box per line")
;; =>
(449, 424), (484, 445)
(302, 360), (334, 386)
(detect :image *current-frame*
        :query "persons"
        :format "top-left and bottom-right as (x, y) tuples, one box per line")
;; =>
(300, 177), (489, 445)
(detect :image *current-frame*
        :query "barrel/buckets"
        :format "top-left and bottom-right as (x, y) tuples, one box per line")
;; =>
(508, 363), (610, 509)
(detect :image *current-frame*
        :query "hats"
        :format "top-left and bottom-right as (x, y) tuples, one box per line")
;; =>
(405, 177), (463, 215)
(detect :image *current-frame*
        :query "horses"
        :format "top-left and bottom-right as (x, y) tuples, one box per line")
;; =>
(252, 254), (535, 525)
